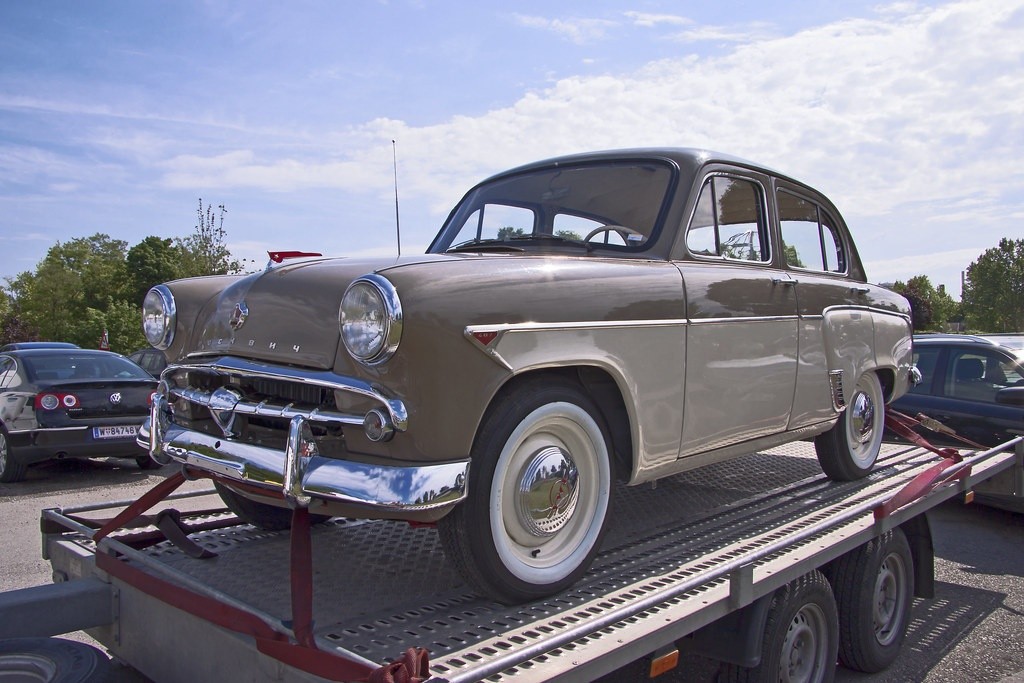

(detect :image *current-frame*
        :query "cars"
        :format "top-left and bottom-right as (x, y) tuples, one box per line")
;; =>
(128, 349), (168, 380)
(0, 349), (161, 483)
(136, 147), (923, 602)
(0, 342), (96, 381)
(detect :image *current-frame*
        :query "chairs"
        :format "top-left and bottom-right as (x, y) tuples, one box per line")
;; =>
(37, 372), (58, 380)
(988, 366), (1008, 387)
(71, 363), (97, 379)
(955, 357), (996, 401)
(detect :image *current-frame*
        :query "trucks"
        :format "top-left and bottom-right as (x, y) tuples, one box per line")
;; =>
(1, 436), (1024, 682)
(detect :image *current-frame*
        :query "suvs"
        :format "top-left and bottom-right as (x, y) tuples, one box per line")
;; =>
(885, 333), (1024, 513)
